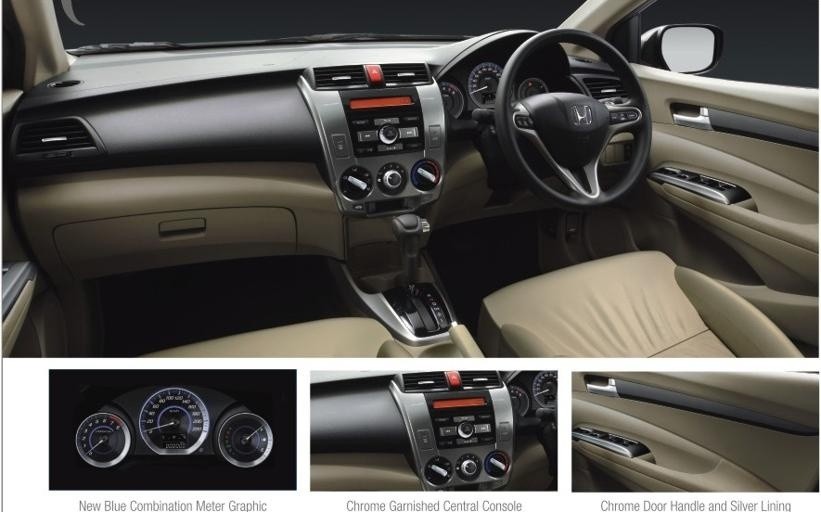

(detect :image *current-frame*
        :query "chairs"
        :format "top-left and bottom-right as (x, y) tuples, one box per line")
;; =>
(476, 250), (819, 359)
(125, 316), (482, 357)
